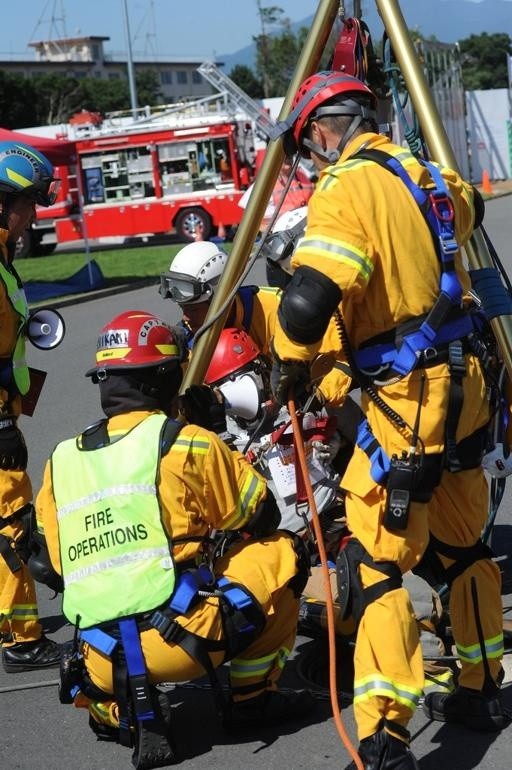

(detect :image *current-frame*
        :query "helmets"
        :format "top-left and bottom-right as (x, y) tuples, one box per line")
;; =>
(84, 310), (183, 385)
(162, 240), (230, 304)
(289, 70), (380, 161)
(202, 327), (261, 385)
(0, 141), (54, 207)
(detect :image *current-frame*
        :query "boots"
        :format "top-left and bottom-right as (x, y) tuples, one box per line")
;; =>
(424, 679), (502, 729)
(220, 689), (315, 731)
(130, 693), (176, 770)
(1, 634), (78, 674)
(344, 733), (416, 768)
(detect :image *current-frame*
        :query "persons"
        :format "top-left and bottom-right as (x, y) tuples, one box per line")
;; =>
(0, 139), (81, 678)
(34, 307), (315, 768)
(262, 66), (512, 767)
(151, 233), (367, 636)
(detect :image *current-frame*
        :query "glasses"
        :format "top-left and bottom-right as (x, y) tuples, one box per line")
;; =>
(157, 270), (204, 303)
(35, 177), (63, 206)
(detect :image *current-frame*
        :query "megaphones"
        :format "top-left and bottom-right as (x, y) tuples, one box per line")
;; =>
(219, 374), (259, 422)
(26, 307), (65, 350)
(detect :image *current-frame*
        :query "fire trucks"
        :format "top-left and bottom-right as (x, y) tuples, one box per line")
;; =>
(10, 94), (271, 260)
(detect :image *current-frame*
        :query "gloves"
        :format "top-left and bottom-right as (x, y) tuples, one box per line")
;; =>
(178, 383), (228, 434)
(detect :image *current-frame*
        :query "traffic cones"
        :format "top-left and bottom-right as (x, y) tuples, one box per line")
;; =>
(481, 170), (493, 194)
(216, 221), (227, 239)
(194, 226), (204, 243)
